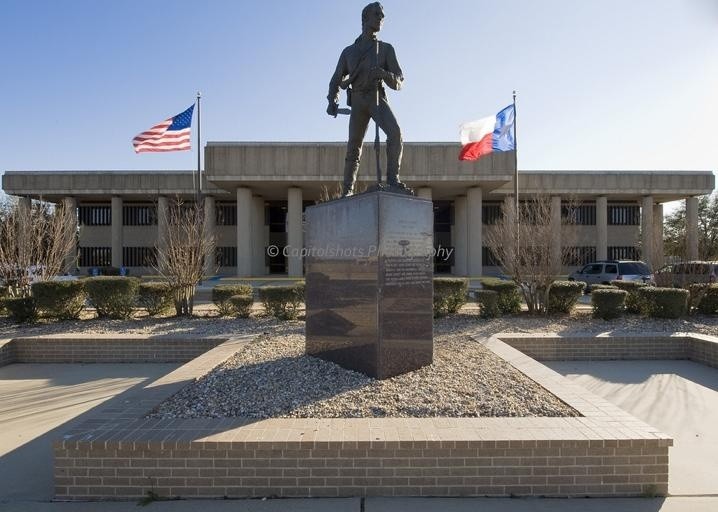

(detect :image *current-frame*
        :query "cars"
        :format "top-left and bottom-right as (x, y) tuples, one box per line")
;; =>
(0, 263), (79, 288)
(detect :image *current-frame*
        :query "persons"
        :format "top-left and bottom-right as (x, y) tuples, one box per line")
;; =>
(326, 1), (414, 199)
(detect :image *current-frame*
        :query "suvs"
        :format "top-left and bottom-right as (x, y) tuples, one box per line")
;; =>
(568, 258), (656, 292)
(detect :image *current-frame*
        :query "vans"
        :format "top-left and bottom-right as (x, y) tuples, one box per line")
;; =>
(650, 260), (717, 288)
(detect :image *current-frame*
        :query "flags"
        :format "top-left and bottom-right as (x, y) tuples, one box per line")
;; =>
(132, 102), (195, 154)
(458, 104), (515, 161)
